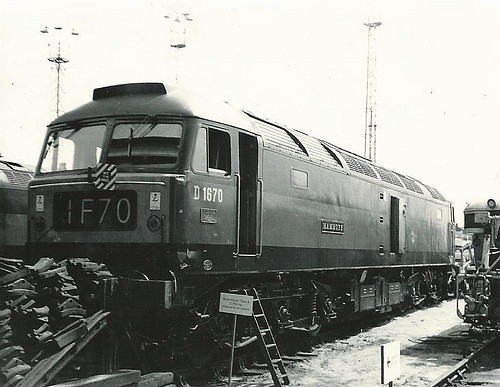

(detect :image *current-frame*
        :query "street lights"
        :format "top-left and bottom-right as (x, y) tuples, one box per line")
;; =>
(40, 24), (79, 172)
(363, 21), (382, 162)
(162, 11), (194, 81)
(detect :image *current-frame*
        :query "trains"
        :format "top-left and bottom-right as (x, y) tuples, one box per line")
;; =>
(0, 159), (65, 264)
(25, 81), (472, 370)
(455, 198), (500, 336)
